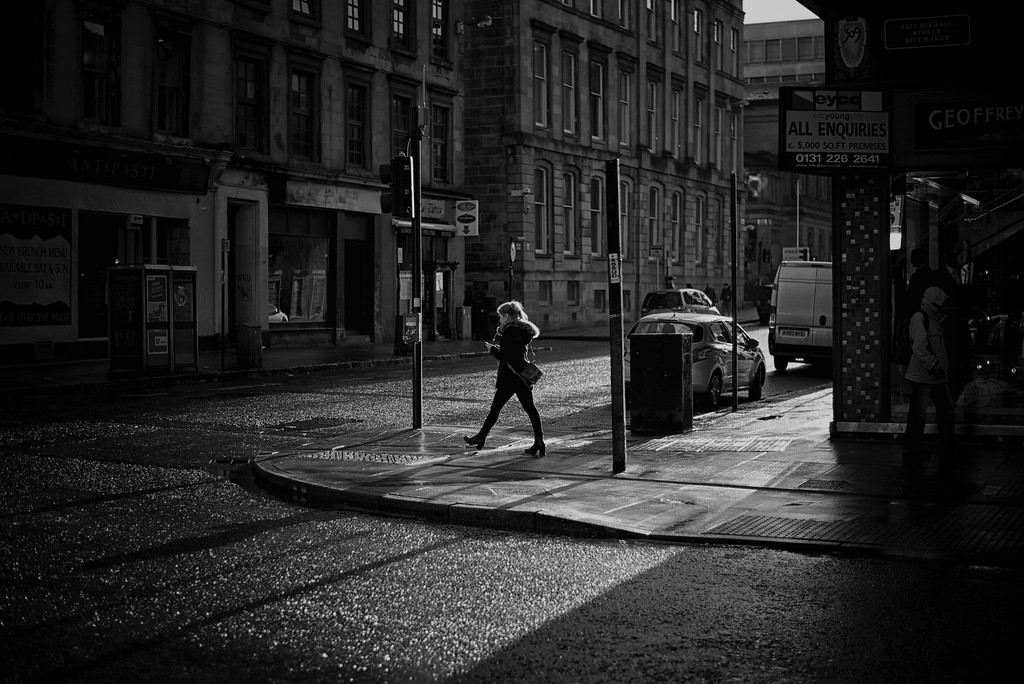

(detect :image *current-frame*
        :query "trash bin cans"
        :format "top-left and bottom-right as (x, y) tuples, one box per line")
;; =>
(630, 333), (693, 436)
(394, 315), (418, 356)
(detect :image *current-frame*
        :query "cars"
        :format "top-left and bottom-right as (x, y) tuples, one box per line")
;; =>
(640, 288), (720, 316)
(267, 304), (289, 323)
(624, 312), (766, 408)
(756, 285), (775, 324)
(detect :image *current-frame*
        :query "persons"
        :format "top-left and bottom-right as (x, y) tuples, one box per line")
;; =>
(899, 284), (978, 502)
(665, 276), (732, 315)
(901, 247), (938, 365)
(463, 298), (547, 458)
(310, 305), (323, 322)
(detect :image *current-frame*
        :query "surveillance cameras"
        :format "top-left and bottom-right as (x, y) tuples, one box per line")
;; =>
(524, 188), (530, 196)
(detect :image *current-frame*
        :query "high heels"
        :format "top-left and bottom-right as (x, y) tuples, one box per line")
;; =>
(525, 441), (546, 456)
(464, 433), (486, 450)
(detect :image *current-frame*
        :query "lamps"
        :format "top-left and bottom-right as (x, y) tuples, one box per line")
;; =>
(455, 15), (492, 35)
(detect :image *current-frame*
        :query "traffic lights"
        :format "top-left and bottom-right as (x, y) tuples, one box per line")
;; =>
(379, 156), (415, 220)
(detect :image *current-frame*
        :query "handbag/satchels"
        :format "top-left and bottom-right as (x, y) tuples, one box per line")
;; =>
(520, 362), (543, 387)
(894, 310), (935, 364)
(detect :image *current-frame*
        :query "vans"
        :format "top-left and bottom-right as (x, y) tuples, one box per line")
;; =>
(767, 261), (834, 371)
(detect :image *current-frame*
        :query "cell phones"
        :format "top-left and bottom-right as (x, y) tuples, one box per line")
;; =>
(485, 342), (488, 344)
